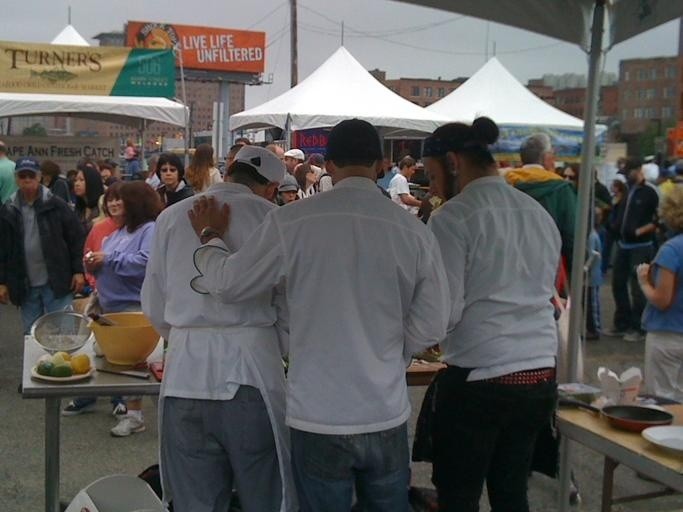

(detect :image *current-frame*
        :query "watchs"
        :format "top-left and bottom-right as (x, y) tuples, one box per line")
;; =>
(201, 227), (223, 243)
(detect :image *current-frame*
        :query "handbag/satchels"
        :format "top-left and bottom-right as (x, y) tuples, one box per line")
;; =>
(612, 182), (655, 270)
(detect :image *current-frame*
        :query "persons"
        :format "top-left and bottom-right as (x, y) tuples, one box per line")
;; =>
(265, 145), (284, 156)
(581, 229), (601, 341)
(294, 163), (318, 198)
(185, 144), (222, 194)
(562, 163), (580, 190)
(39, 160), (69, 202)
(75, 166), (105, 235)
(637, 178), (682, 406)
(142, 143), (296, 512)
(595, 171), (609, 201)
(609, 180), (630, 228)
(411, 117), (562, 512)
(389, 155), (422, 213)
(617, 152), (682, 182)
(145, 154), (161, 190)
(67, 171), (80, 208)
(76, 157), (100, 172)
(63, 180), (126, 420)
(0, 140), (17, 204)
(600, 157), (659, 344)
(125, 139), (138, 175)
(503, 132), (591, 280)
(87, 180), (164, 437)
(156, 154), (196, 209)
(0, 155), (86, 394)
(274, 176), (298, 206)
(284, 148), (305, 175)
(307, 153), (332, 192)
(188, 119), (448, 512)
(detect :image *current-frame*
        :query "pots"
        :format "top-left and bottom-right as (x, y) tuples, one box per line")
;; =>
(560, 400), (674, 432)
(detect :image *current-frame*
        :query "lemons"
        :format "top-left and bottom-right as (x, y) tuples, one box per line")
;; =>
(70, 354), (90, 373)
(53, 351), (70, 365)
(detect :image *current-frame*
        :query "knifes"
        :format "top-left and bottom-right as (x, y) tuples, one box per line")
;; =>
(99, 367), (149, 377)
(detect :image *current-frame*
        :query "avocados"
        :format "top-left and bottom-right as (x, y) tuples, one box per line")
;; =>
(50, 364), (72, 377)
(37, 362), (54, 375)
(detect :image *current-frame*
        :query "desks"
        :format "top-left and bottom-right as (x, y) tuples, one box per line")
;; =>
(22, 333), (447, 512)
(554, 402), (683, 511)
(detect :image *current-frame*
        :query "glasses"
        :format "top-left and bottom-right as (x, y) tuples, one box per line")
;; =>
(562, 174), (575, 179)
(160, 168), (176, 172)
(17, 172), (36, 179)
(307, 169), (314, 173)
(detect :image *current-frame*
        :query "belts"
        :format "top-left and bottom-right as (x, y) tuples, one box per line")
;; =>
(484, 369), (553, 384)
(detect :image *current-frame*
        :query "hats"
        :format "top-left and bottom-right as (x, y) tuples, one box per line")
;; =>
(616, 157), (641, 174)
(278, 175), (298, 192)
(15, 156), (40, 173)
(284, 148), (304, 160)
(233, 144), (286, 190)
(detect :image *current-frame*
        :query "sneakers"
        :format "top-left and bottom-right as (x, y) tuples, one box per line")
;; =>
(602, 327), (624, 337)
(62, 400), (96, 416)
(623, 328), (645, 342)
(112, 401), (127, 418)
(110, 410), (146, 436)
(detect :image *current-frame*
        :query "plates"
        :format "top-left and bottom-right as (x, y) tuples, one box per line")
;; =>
(558, 382), (601, 398)
(640, 425), (683, 450)
(31, 366), (96, 381)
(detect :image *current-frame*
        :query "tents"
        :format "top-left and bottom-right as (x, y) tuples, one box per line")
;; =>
(0, 24), (190, 169)
(228, 45), (445, 144)
(388, 55), (610, 142)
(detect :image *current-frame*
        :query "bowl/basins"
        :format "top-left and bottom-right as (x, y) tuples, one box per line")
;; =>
(30, 309), (93, 355)
(88, 312), (161, 365)
